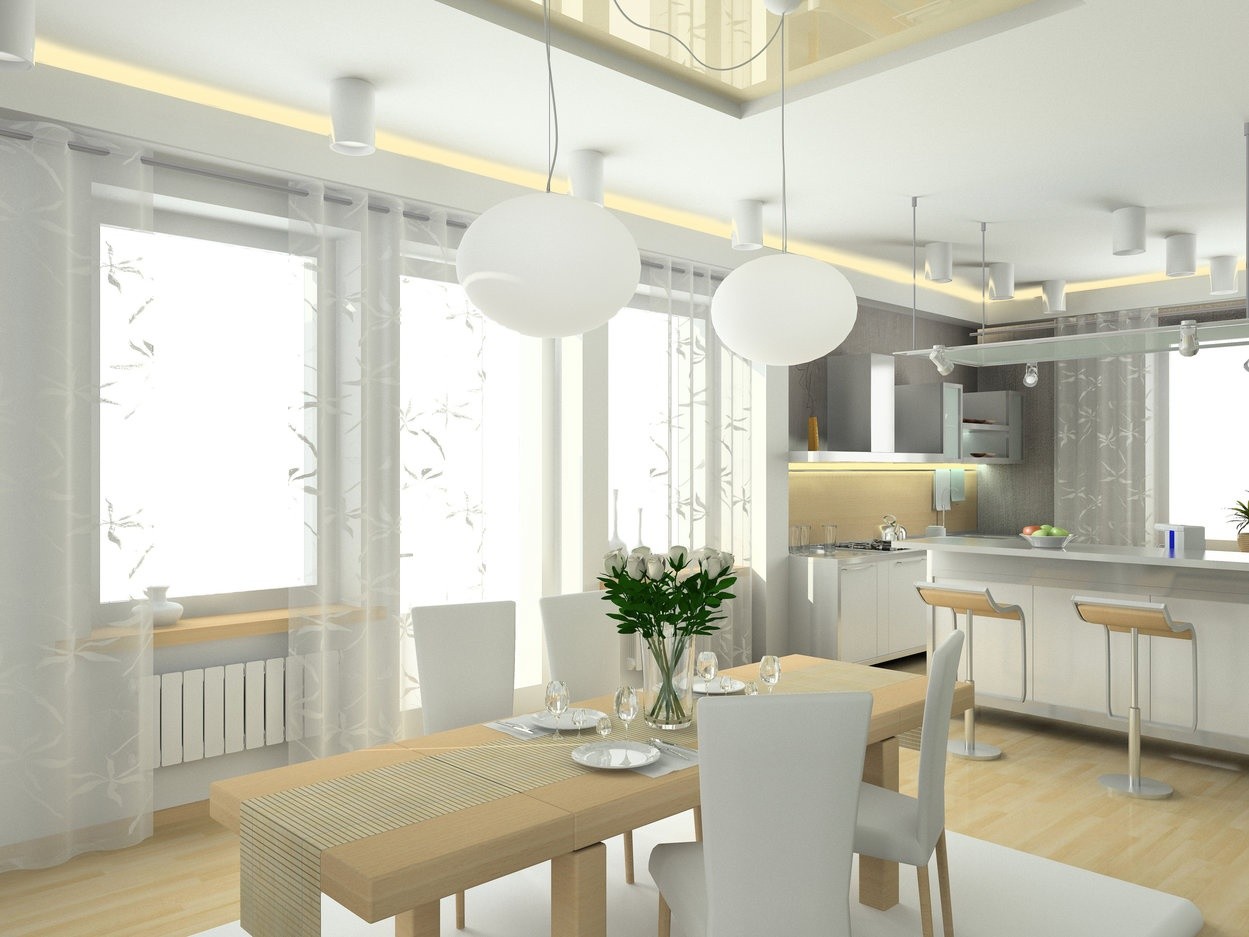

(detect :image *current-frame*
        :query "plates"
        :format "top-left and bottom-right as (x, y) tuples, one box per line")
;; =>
(571, 741), (660, 767)
(691, 678), (743, 695)
(532, 708), (606, 730)
(1018, 534), (1078, 547)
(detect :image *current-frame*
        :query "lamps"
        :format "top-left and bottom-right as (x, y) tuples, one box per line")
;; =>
(732, 200), (764, 251)
(709, 0), (859, 369)
(1209, 254), (1238, 294)
(987, 262), (1016, 301)
(455, 0), (643, 335)
(1166, 233), (1197, 279)
(1110, 204), (1147, 257)
(1021, 360), (1039, 387)
(565, 149), (603, 208)
(1179, 319), (1199, 357)
(925, 242), (954, 283)
(1, 0), (40, 68)
(327, 76), (380, 158)
(929, 343), (954, 375)
(1040, 277), (1066, 315)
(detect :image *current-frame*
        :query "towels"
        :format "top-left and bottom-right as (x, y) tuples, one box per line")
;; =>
(933, 468), (951, 512)
(948, 467), (966, 501)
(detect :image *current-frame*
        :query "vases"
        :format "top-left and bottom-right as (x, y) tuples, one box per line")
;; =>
(639, 627), (696, 731)
(808, 416), (819, 451)
(130, 585), (183, 627)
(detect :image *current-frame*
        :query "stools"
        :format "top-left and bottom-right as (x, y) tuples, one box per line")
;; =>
(1070, 593), (1199, 801)
(912, 581), (1027, 762)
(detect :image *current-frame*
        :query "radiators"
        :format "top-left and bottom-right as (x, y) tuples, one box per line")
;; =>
(134, 657), (325, 772)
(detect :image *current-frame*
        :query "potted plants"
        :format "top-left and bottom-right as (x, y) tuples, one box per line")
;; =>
(1226, 500), (1249, 552)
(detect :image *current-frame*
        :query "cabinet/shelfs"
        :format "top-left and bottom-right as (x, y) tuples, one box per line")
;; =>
(895, 382), (1023, 466)
(890, 554), (927, 660)
(789, 553), (878, 666)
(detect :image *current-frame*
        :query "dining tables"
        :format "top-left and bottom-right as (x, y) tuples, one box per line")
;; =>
(209, 653), (975, 937)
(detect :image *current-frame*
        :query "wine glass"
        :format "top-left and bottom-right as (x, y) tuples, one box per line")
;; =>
(596, 717), (611, 740)
(572, 709), (586, 742)
(545, 681), (570, 739)
(614, 686), (638, 742)
(698, 650), (782, 697)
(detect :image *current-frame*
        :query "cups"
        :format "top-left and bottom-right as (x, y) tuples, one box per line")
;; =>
(925, 525), (946, 539)
(822, 524), (837, 557)
(790, 525), (811, 556)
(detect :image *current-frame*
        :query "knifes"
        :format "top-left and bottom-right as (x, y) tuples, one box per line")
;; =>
(496, 722), (533, 735)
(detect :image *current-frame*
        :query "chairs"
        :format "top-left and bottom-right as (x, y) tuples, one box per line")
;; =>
(411, 600), (518, 928)
(851, 629), (966, 937)
(540, 588), (703, 884)
(648, 691), (875, 937)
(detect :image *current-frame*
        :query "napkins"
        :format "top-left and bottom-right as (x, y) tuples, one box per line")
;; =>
(483, 714), (559, 740)
(626, 740), (700, 779)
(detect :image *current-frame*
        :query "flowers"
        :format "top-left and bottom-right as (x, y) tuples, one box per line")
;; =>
(597, 545), (740, 725)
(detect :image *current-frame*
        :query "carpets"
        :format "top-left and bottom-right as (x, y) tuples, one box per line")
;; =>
(188, 807), (1205, 937)
